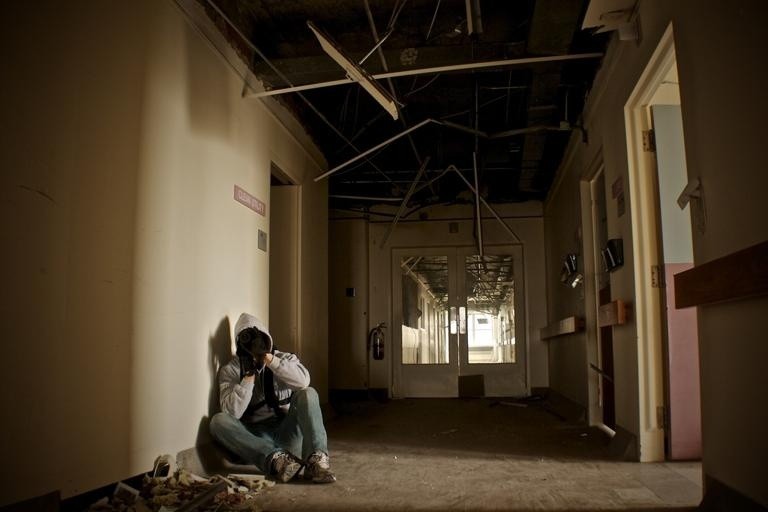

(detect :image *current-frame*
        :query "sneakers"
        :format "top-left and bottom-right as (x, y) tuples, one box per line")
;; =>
(266, 449), (304, 483)
(305, 449), (336, 483)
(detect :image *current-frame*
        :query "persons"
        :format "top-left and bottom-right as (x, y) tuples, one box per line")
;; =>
(209, 313), (337, 484)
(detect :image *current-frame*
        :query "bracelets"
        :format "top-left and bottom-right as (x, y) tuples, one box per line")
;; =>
(269, 355), (274, 365)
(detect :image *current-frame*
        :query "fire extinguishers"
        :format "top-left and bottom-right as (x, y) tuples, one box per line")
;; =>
(367, 322), (387, 360)
